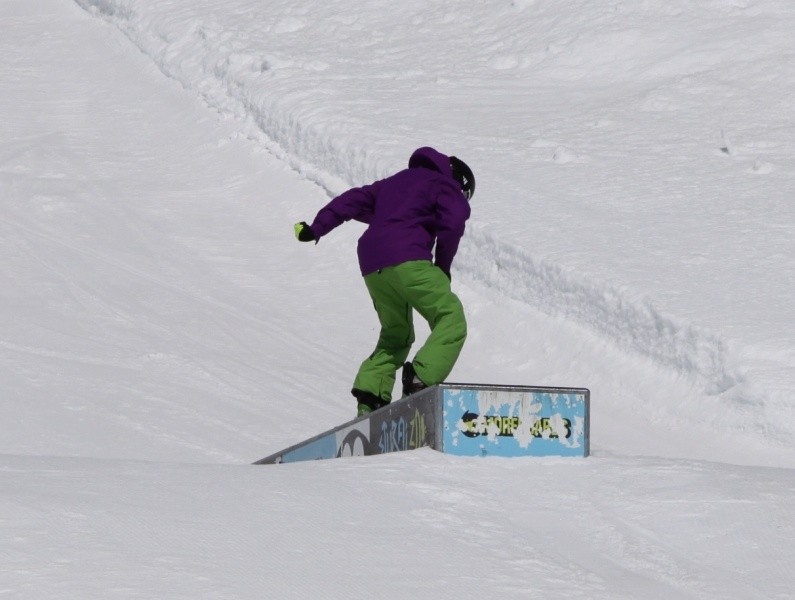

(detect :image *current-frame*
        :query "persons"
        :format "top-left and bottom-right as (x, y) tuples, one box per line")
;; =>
(293, 145), (476, 418)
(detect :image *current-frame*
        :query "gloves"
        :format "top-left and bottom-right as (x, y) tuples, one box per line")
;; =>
(295, 221), (314, 242)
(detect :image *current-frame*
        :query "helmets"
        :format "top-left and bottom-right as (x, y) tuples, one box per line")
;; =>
(449, 156), (475, 201)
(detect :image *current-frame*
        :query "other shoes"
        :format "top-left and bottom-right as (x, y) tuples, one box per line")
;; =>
(357, 395), (389, 418)
(402, 361), (429, 400)
